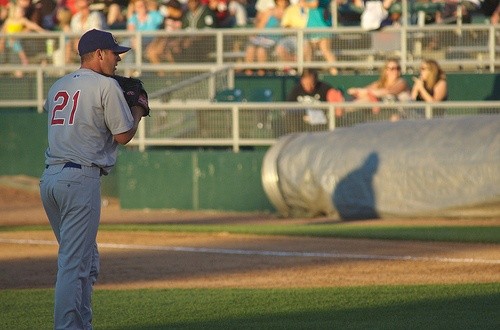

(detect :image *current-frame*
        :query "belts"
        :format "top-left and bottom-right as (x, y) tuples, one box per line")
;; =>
(45, 162), (81, 168)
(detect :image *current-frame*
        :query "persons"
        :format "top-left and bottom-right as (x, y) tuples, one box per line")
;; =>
(0, 0), (500, 136)
(38, 28), (150, 330)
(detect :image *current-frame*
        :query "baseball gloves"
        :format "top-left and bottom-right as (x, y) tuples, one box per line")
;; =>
(112, 75), (153, 118)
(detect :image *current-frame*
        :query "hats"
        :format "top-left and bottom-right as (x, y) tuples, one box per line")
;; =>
(78, 28), (131, 55)
